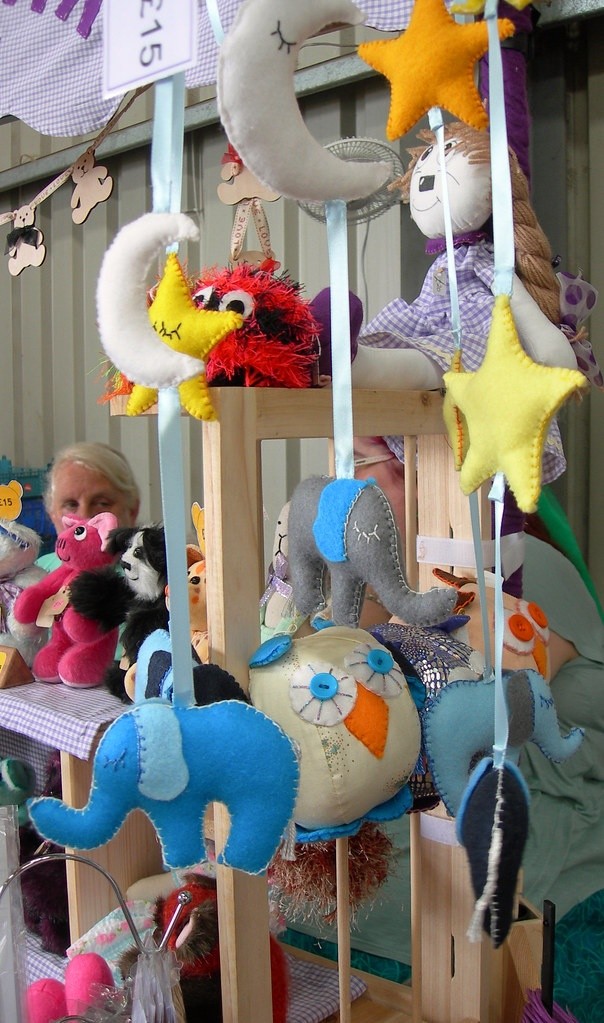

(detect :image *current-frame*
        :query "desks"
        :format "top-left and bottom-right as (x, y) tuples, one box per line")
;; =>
(1, 672), (162, 956)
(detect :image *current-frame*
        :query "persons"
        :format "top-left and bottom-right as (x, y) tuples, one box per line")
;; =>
(37, 441), (138, 600)
(26, 821), (394, 1023)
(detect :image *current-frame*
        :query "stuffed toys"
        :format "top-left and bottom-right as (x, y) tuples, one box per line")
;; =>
(124, 501), (207, 704)
(66, 524), (167, 705)
(0, 462), (123, 689)
(153, 871), (290, 1023)
(305, 119), (604, 488)
